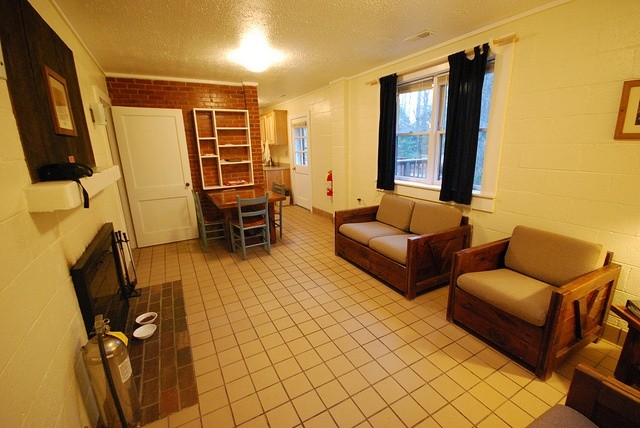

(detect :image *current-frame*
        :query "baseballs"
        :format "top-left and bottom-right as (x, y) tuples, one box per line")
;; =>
(80, 314), (143, 428)
(326, 170), (333, 196)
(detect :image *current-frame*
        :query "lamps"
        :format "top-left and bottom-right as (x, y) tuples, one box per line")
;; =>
(193, 192), (226, 252)
(229, 193), (270, 261)
(273, 181), (284, 240)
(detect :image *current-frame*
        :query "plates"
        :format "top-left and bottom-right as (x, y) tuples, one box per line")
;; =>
(260, 110), (289, 145)
(193, 108), (255, 190)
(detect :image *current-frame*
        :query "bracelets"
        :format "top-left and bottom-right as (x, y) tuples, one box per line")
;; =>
(135, 312), (158, 325)
(133, 324), (156, 340)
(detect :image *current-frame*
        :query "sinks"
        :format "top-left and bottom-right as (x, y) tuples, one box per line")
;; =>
(445, 223), (622, 380)
(613, 80), (640, 140)
(524, 364), (639, 428)
(335, 194), (473, 301)
